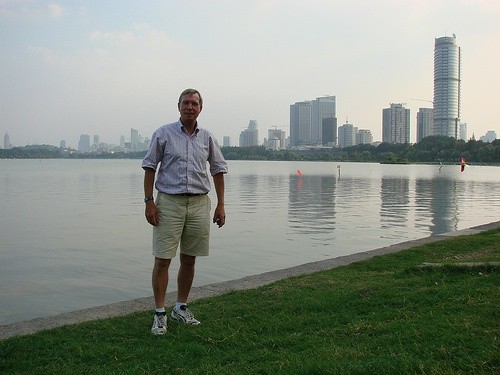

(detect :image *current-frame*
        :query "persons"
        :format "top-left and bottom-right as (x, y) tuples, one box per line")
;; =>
(142, 89), (228, 335)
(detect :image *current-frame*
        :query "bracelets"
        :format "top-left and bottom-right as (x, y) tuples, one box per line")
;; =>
(143, 196), (153, 204)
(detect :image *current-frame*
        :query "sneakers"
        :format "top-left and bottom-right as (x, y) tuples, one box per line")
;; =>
(151, 312), (167, 335)
(170, 307), (201, 325)
